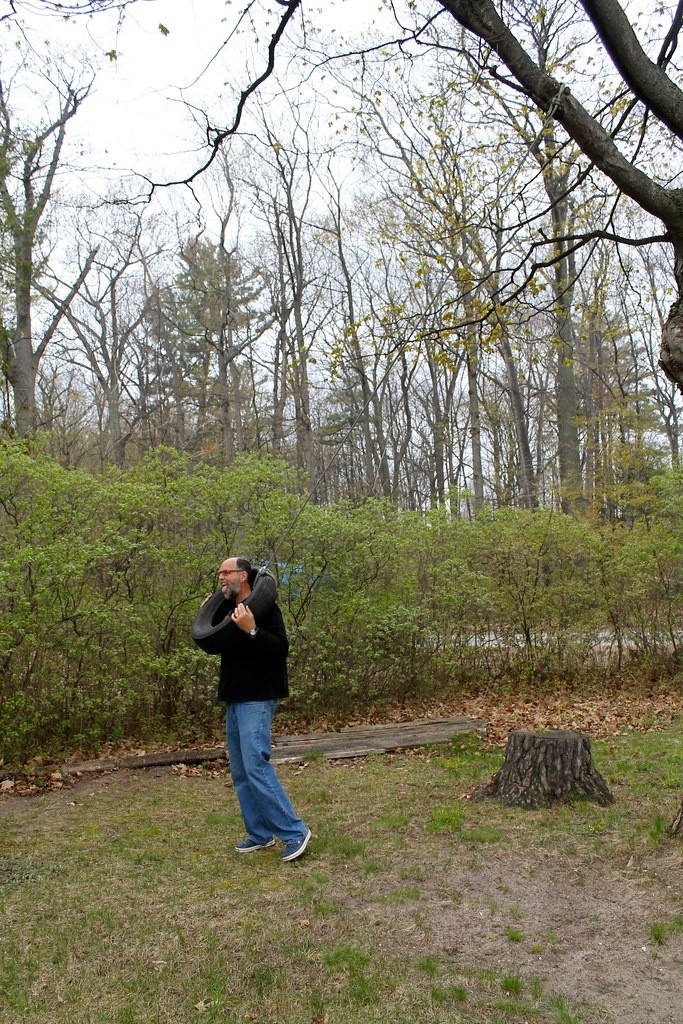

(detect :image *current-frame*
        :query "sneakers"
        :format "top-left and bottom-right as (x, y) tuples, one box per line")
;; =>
(282, 828), (311, 862)
(235, 837), (275, 852)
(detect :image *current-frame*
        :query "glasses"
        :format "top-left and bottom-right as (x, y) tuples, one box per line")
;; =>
(217, 570), (243, 576)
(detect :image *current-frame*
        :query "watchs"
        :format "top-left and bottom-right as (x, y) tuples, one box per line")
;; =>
(248, 627), (259, 637)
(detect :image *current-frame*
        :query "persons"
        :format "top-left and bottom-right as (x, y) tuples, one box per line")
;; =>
(199, 557), (311, 863)
